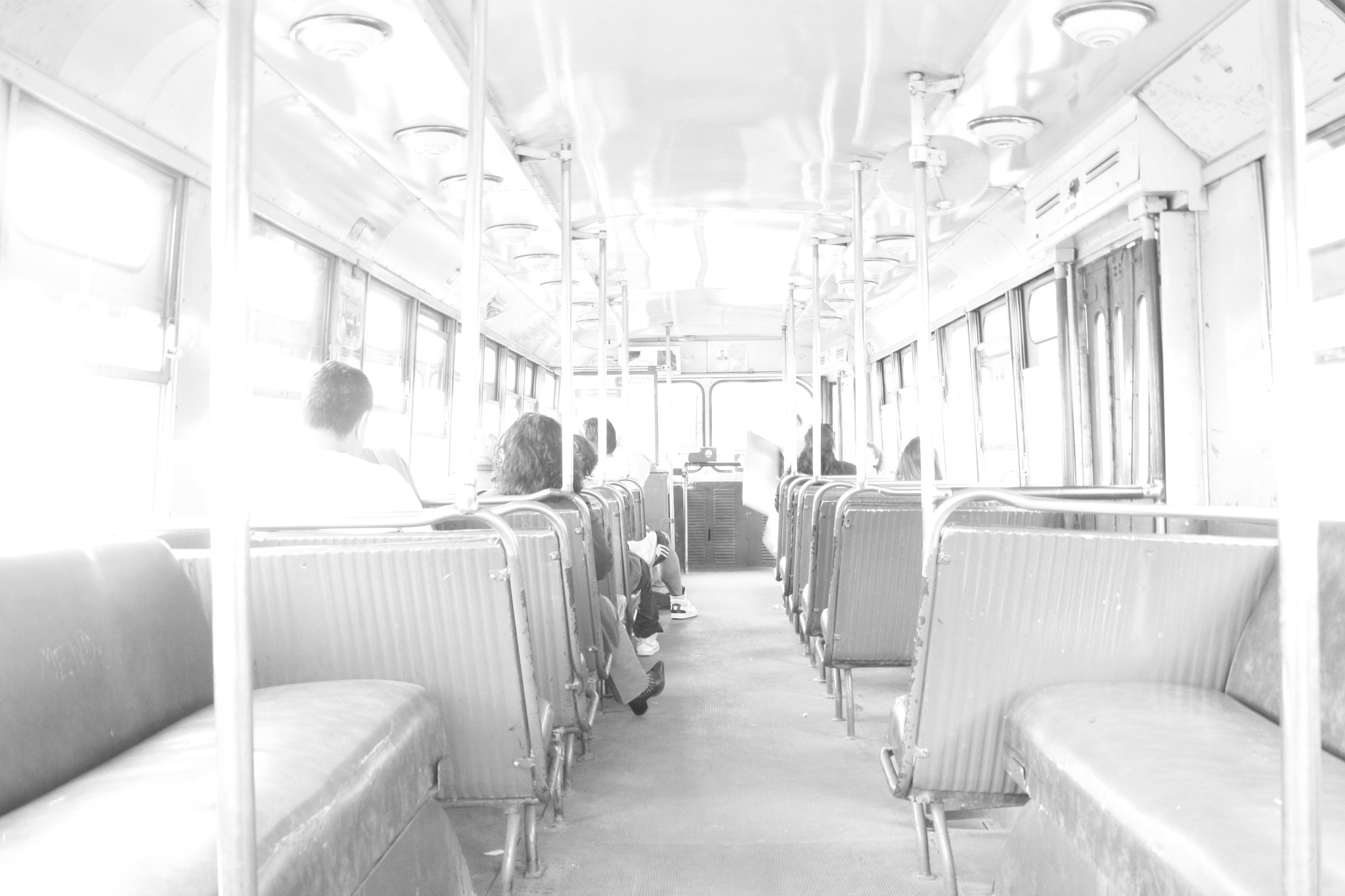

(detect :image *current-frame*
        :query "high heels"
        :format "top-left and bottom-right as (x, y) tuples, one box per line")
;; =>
(628, 660), (665, 715)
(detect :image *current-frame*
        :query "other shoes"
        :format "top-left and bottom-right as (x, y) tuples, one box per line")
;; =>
(652, 590), (671, 608)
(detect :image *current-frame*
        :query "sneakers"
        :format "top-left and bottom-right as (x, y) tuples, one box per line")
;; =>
(670, 586), (698, 619)
(636, 631), (659, 655)
(627, 530), (658, 567)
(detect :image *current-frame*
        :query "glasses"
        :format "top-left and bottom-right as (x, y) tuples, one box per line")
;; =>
(807, 423), (834, 442)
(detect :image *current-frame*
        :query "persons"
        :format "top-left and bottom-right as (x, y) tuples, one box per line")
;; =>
(867, 442), (896, 475)
(775, 424), (857, 513)
(778, 414), (804, 476)
(303, 359), (423, 504)
(478, 411), (664, 715)
(895, 436), (943, 481)
(583, 418), (698, 619)
(645, 525), (665, 587)
(574, 434), (665, 656)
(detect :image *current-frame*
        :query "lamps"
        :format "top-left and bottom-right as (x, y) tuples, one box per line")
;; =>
(967, 117), (1045, 148)
(290, 15), (556, 274)
(819, 234), (912, 331)
(1055, 1), (1156, 49)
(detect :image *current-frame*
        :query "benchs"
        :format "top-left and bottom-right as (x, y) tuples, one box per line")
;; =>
(0, 472), (1345, 896)
